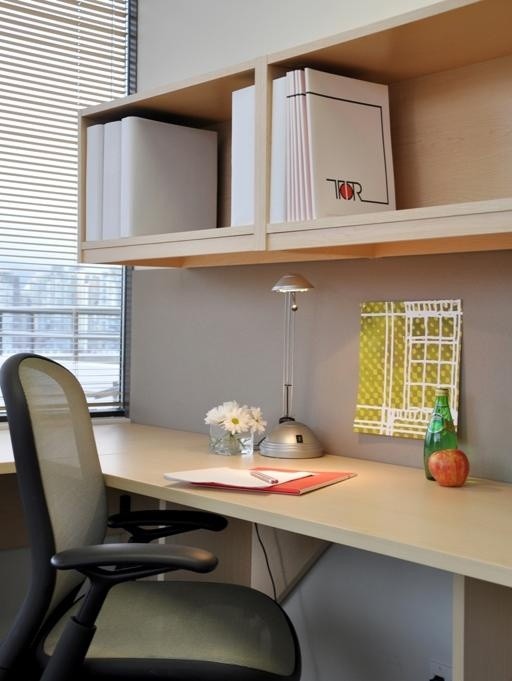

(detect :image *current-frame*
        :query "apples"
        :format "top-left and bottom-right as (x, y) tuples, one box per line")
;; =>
(429, 450), (469, 487)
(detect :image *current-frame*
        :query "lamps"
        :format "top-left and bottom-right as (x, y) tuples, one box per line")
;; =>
(259, 274), (324, 457)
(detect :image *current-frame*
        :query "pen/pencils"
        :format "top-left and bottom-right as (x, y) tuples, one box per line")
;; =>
(251, 471), (278, 484)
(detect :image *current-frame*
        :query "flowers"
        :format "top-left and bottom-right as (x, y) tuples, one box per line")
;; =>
(205, 399), (268, 454)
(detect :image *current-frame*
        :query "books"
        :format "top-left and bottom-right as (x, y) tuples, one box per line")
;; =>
(230, 68), (398, 228)
(163, 466), (358, 496)
(83, 117), (218, 238)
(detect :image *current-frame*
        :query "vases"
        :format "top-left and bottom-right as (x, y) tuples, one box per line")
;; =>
(209, 424), (254, 453)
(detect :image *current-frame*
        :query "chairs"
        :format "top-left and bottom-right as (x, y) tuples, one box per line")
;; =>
(1, 352), (301, 680)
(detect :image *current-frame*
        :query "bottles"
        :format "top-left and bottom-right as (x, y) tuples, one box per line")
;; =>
(423, 387), (458, 481)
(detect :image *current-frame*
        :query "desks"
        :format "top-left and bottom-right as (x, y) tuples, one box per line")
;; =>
(3, 416), (512, 680)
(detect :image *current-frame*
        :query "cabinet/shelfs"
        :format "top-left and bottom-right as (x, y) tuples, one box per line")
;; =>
(78, 1), (511, 269)
